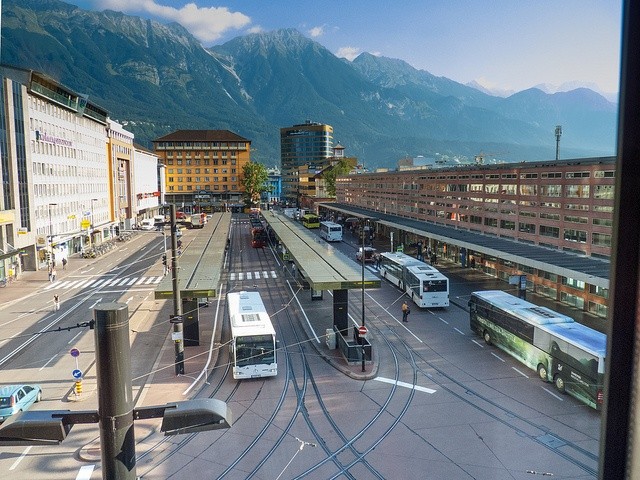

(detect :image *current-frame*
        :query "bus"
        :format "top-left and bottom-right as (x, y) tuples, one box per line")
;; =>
(467, 290), (608, 412)
(319, 220), (343, 242)
(250, 226), (266, 248)
(379, 251), (450, 308)
(302, 213), (321, 229)
(227, 290), (278, 380)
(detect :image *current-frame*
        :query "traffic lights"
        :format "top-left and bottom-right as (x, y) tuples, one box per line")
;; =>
(116, 226), (119, 235)
(162, 255), (166, 265)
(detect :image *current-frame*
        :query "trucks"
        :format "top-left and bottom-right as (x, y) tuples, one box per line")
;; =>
(141, 218), (155, 230)
(191, 213), (207, 228)
(154, 215), (165, 226)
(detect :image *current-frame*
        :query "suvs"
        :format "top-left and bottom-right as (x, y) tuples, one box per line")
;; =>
(356, 247), (376, 263)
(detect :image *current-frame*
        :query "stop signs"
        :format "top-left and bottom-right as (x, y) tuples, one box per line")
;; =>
(358, 326), (367, 334)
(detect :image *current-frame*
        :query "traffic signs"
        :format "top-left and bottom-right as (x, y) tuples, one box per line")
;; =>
(0, 302), (232, 480)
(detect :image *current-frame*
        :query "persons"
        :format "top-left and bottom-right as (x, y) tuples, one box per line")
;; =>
(227, 238), (230, 247)
(470, 256), (476, 274)
(53, 294), (60, 313)
(53, 268), (56, 282)
(406, 237), (439, 266)
(48, 268), (53, 284)
(62, 258), (67, 269)
(401, 301), (410, 322)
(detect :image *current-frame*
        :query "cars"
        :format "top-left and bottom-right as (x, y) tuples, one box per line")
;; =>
(0, 384), (43, 423)
(165, 215), (171, 223)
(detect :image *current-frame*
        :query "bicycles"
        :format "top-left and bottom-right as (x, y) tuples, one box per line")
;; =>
(0, 280), (6, 288)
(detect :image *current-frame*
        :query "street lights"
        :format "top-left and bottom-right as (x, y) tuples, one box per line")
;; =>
(155, 204), (194, 376)
(91, 199), (98, 252)
(48, 203), (57, 270)
(345, 216), (379, 372)
